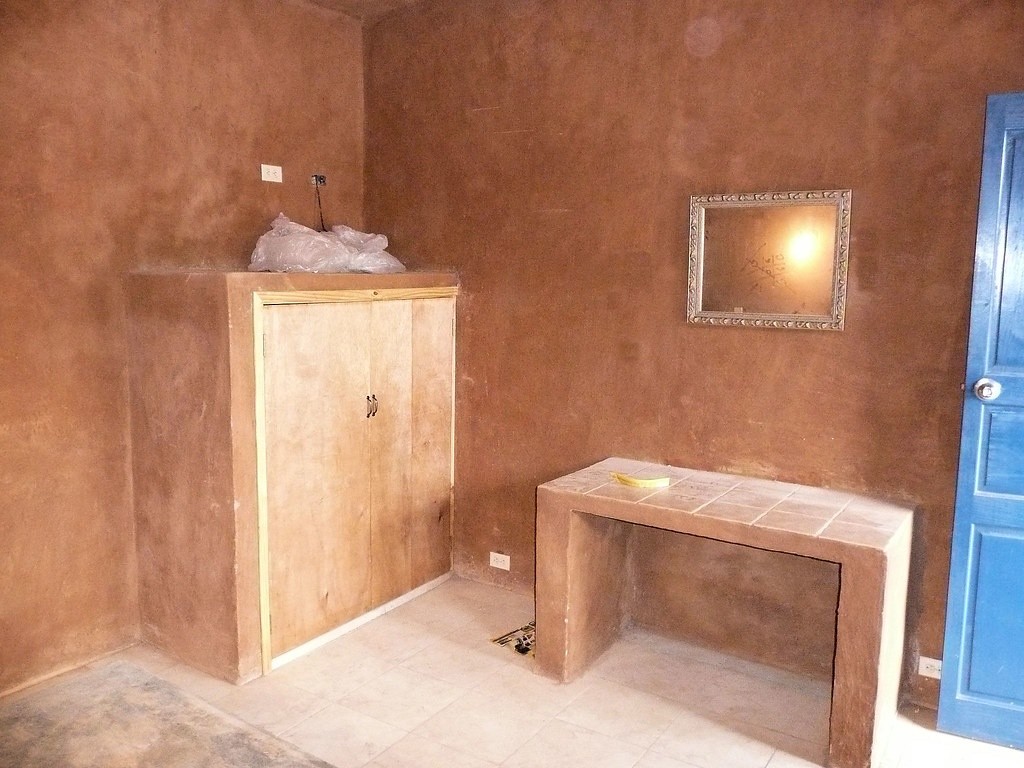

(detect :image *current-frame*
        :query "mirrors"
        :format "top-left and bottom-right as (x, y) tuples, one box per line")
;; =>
(684, 188), (852, 332)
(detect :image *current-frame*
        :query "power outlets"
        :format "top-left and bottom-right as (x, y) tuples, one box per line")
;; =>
(918, 655), (942, 680)
(261, 163), (282, 183)
(311, 174), (328, 189)
(489, 551), (510, 572)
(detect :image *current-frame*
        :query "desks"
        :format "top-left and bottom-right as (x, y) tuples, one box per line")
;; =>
(533, 456), (914, 768)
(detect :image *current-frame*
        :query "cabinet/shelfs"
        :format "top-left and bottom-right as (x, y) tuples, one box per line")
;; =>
(123, 267), (459, 684)
